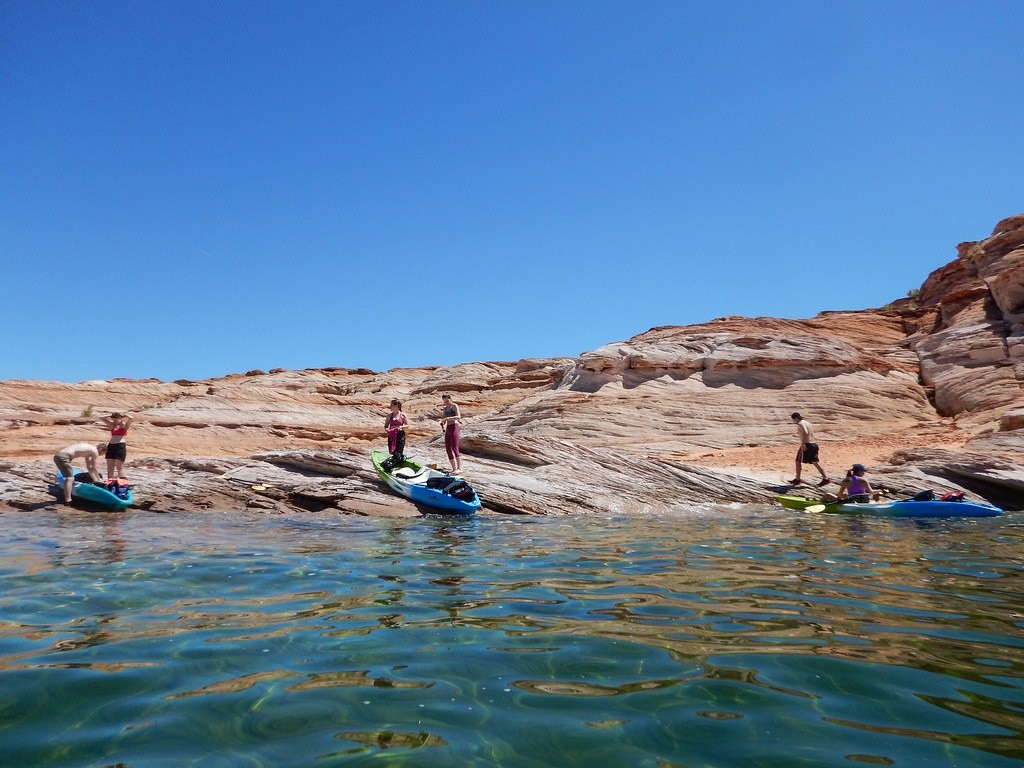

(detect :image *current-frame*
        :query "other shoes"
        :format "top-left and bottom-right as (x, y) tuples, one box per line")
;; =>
(817, 478), (831, 487)
(788, 479), (801, 484)
(64, 500), (82, 506)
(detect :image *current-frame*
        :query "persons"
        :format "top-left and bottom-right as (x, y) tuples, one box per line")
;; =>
(788, 413), (830, 486)
(384, 398), (409, 455)
(53, 443), (107, 506)
(99, 413), (134, 479)
(819, 463), (883, 503)
(442, 394), (462, 474)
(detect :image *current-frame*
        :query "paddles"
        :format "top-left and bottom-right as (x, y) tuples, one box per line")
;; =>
(895, 489), (933, 502)
(215, 476), (273, 491)
(805, 489), (889, 514)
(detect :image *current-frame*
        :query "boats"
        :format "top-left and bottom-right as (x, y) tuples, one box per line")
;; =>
(371, 447), (483, 513)
(777, 491), (1005, 519)
(57, 465), (137, 510)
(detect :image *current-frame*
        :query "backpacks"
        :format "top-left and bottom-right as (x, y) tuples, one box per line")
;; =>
(940, 490), (967, 502)
(381, 450), (407, 471)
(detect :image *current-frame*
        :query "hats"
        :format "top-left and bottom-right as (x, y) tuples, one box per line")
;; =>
(791, 412), (804, 418)
(853, 463), (866, 471)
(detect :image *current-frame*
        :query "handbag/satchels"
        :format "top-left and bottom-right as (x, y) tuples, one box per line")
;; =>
(105, 484), (129, 498)
